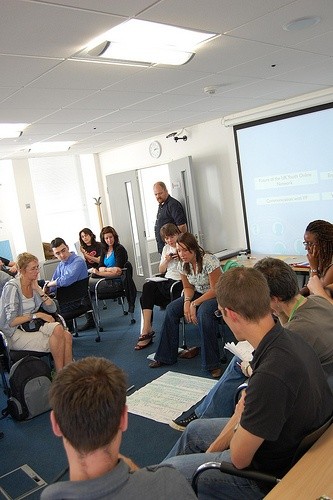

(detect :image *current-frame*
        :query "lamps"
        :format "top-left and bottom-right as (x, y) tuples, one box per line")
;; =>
(167, 132), (187, 142)
(88, 41), (196, 66)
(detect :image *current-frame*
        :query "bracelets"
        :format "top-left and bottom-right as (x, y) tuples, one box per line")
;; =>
(44, 282), (50, 288)
(41, 293), (45, 297)
(30, 313), (37, 319)
(309, 269), (317, 273)
(44, 297), (49, 302)
(192, 301), (197, 307)
(184, 297), (191, 302)
(243, 364), (251, 377)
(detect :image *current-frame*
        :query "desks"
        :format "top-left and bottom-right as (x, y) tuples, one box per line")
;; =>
(262, 424), (333, 500)
(220, 254), (310, 288)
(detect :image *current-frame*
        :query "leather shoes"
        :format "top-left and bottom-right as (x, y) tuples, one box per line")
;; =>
(212, 368), (223, 379)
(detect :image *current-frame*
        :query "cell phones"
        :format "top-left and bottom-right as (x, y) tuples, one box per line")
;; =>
(169, 253), (174, 257)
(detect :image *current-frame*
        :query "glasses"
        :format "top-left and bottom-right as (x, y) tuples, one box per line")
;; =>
(26, 266), (42, 271)
(215, 307), (229, 317)
(303, 241), (319, 247)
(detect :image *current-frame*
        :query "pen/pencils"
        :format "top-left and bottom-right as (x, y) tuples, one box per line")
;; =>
(126, 384), (135, 392)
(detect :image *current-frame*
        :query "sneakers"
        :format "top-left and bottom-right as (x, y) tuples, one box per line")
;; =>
(168, 412), (198, 432)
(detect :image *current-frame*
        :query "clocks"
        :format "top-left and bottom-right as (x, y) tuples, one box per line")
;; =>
(149, 140), (161, 159)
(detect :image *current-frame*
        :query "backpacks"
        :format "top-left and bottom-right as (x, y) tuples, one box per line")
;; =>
(0, 356), (54, 423)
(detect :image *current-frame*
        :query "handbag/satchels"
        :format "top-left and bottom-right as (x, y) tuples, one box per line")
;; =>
(19, 308), (57, 332)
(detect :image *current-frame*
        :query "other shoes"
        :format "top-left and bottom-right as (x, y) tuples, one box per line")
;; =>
(139, 330), (155, 340)
(78, 318), (98, 331)
(134, 338), (152, 350)
(149, 360), (162, 367)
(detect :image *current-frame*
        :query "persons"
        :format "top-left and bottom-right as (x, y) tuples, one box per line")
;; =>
(78, 228), (102, 270)
(152, 181), (187, 255)
(41, 357), (199, 500)
(133, 224), (181, 350)
(0, 256), (18, 297)
(0, 252), (73, 377)
(42, 237), (88, 334)
(170, 257), (333, 430)
(159, 265), (333, 500)
(78, 226), (128, 332)
(147, 232), (225, 378)
(298, 219), (333, 303)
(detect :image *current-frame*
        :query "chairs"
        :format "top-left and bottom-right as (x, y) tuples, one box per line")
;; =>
(150, 273), (232, 363)
(0, 274), (134, 368)
(192, 382), (333, 497)
